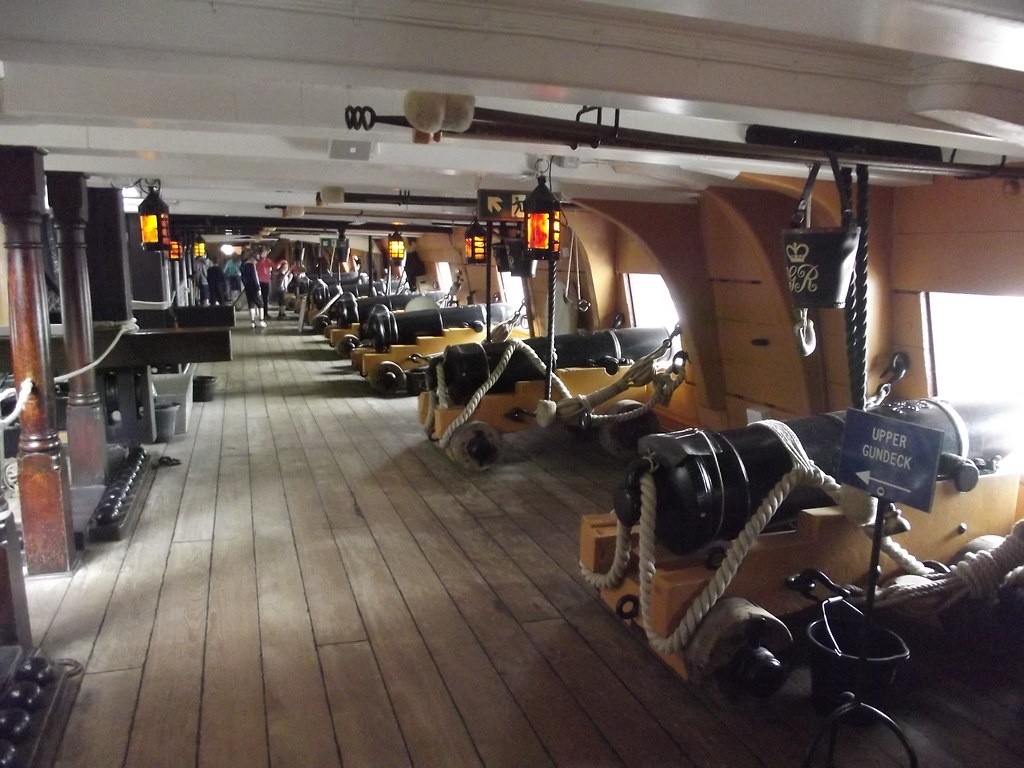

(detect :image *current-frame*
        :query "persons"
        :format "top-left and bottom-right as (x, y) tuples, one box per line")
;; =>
(189, 245), (315, 332)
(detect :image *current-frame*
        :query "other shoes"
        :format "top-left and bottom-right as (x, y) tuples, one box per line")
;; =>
(278, 313), (291, 320)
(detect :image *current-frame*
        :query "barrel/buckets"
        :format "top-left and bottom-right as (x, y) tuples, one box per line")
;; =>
(193, 376), (216, 401)
(155, 403), (180, 441)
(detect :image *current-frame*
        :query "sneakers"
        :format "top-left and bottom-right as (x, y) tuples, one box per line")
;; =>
(259, 321), (267, 328)
(250, 323), (256, 328)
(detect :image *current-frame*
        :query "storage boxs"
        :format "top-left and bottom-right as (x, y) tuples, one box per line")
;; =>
(334, 236), (352, 262)
(782, 148), (863, 310)
(491, 220), (512, 274)
(503, 220), (539, 279)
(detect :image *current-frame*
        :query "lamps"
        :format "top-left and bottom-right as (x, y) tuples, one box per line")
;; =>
(521, 157), (563, 261)
(192, 232), (207, 261)
(389, 228), (405, 261)
(169, 235), (184, 261)
(464, 209), (490, 265)
(137, 177), (172, 252)
